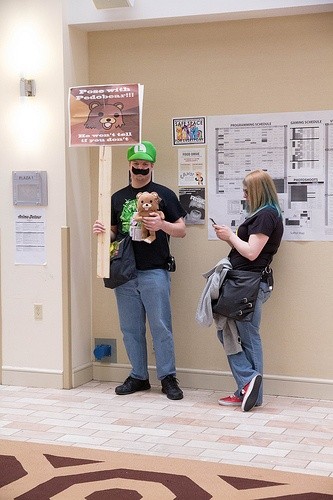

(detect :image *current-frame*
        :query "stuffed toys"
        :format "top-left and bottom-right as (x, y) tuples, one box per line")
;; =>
(135, 191), (165, 244)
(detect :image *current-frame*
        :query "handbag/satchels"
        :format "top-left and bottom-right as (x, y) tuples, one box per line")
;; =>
(210, 269), (262, 322)
(167, 255), (176, 272)
(102, 234), (138, 289)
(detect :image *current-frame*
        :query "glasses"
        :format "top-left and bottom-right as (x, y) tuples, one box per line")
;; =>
(242, 189), (248, 195)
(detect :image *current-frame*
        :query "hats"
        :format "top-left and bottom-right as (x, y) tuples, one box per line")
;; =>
(126, 141), (157, 163)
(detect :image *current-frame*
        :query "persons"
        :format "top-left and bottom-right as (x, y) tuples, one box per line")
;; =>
(213, 170), (284, 413)
(92, 141), (186, 400)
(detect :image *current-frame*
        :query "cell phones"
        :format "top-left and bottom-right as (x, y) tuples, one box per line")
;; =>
(209, 218), (217, 225)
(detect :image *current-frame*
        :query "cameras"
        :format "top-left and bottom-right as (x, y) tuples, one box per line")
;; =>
(167, 256), (176, 272)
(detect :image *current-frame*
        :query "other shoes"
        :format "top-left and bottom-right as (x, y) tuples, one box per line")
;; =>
(242, 374), (263, 411)
(218, 394), (245, 406)
(114, 376), (151, 395)
(161, 375), (184, 400)
